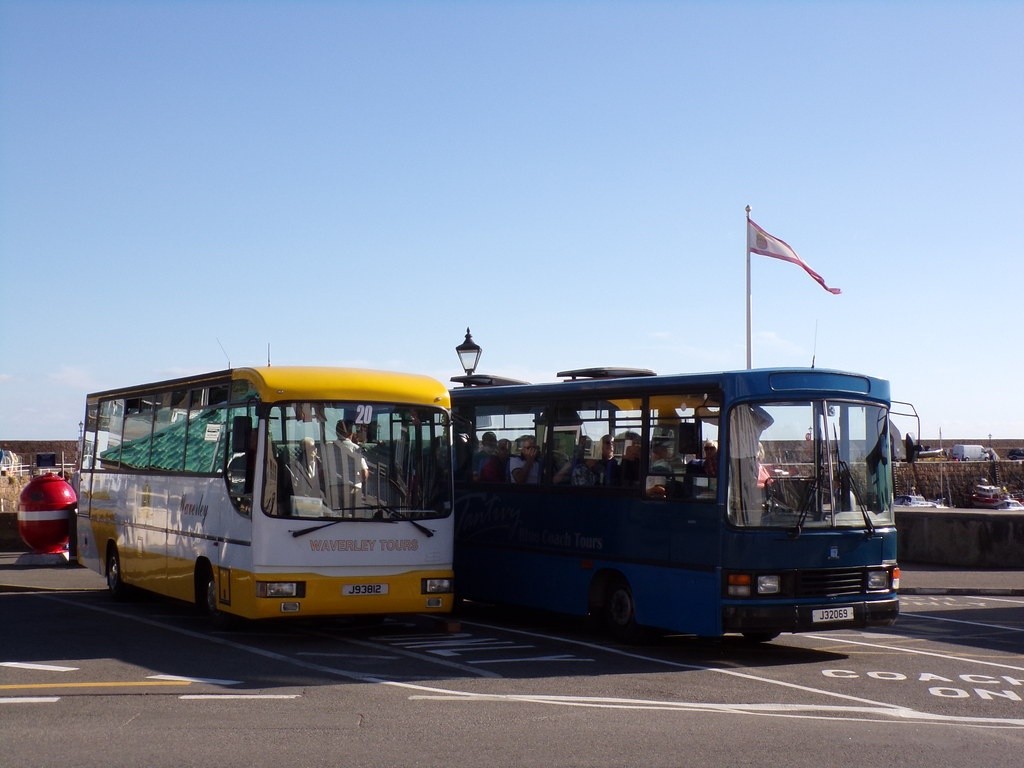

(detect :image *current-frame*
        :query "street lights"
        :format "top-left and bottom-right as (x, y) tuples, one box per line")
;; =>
(79, 421), (84, 459)
(809, 426), (813, 458)
(455, 327), (482, 375)
(988, 434), (992, 460)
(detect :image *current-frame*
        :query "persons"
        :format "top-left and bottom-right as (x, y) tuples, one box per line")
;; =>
(435, 402), (774, 526)
(290, 419), (369, 511)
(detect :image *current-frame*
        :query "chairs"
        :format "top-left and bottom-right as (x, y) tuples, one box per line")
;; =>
(467, 451), (704, 498)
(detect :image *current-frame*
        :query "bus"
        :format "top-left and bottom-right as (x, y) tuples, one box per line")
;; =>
(393, 366), (925, 642)
(76, 365), (455, 627)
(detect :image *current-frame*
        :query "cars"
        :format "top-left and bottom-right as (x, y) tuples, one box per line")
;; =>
(1007, 448), (1024, 460)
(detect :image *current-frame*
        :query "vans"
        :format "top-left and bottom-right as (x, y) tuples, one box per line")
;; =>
(952, 445), (989, 462)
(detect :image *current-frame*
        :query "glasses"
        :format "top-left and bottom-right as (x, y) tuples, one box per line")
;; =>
(704, 446), (716, 451)
(606, 441), (615, 445)
(524, 446), (538, 450)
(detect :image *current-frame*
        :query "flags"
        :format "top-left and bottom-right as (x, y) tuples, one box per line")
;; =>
(747, 217), (842, 294)
